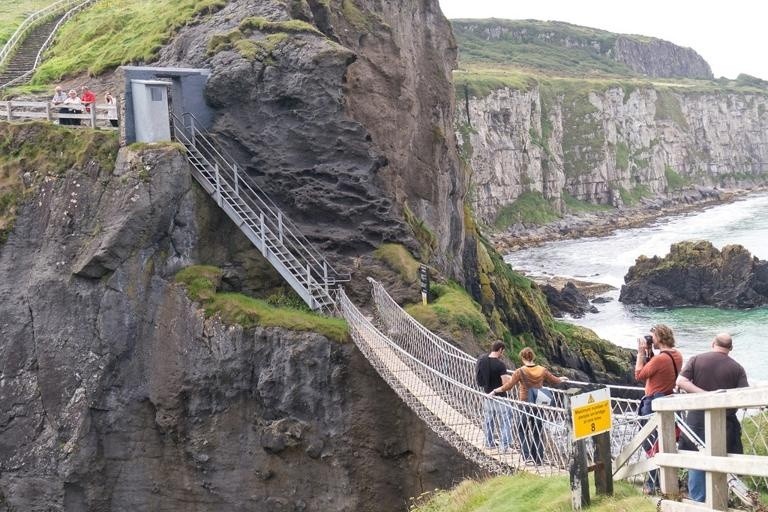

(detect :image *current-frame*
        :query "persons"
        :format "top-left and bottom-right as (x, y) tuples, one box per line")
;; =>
(52, 86), (118, 127)
(676, 333), (749, 503)
(487, 347), (568, 466)
(635, 324), (683, 495)
(475, 340), (517, 452)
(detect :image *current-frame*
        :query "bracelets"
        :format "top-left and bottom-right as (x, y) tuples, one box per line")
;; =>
(494, 389), (496, 394)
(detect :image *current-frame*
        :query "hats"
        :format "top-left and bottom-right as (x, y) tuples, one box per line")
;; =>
(67, 89), (77, 97)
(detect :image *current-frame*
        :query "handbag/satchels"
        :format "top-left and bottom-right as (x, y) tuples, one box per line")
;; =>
(528, 387), (556, 407)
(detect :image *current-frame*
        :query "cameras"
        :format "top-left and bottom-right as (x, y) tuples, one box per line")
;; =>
(644, 336), (653, 344)
